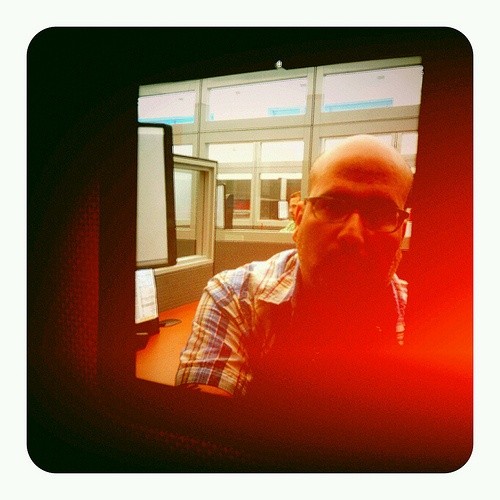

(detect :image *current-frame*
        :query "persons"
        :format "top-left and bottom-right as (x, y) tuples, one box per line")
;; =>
(278, 190), (302, 232)
(175, 132), (414, 405)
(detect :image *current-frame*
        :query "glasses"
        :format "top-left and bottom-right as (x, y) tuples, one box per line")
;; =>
(304, 196), (410, 235)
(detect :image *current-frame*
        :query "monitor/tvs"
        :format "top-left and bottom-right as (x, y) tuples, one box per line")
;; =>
(138, 121), (179, 270)
